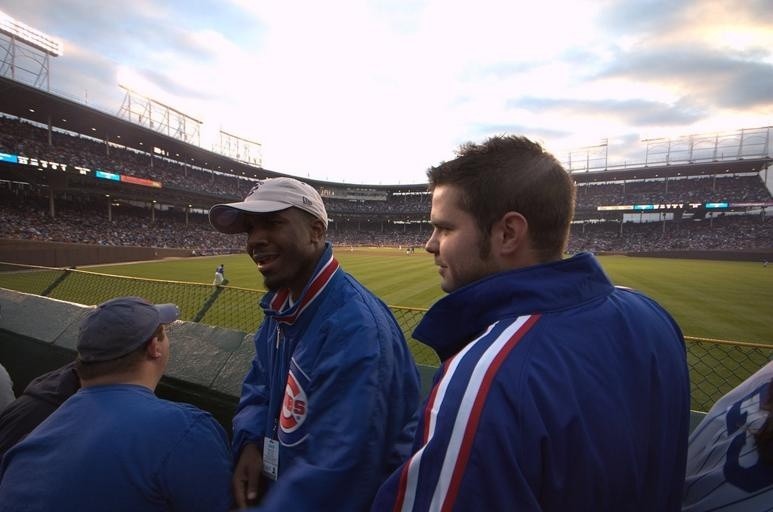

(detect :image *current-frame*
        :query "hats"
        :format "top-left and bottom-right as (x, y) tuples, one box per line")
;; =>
(76, 296), (181, 363)
(208, 177), (328, 235)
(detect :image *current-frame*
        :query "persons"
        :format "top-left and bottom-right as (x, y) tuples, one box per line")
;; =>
(205, 175), (425, 512)
(368, 134), (692, 512)
(0, 111), (252, 257)
(321, 170), (772, 257)
(681, 359), (770, 510)
(0, 292), (238, 509)
(211, 264), (224, 286)
